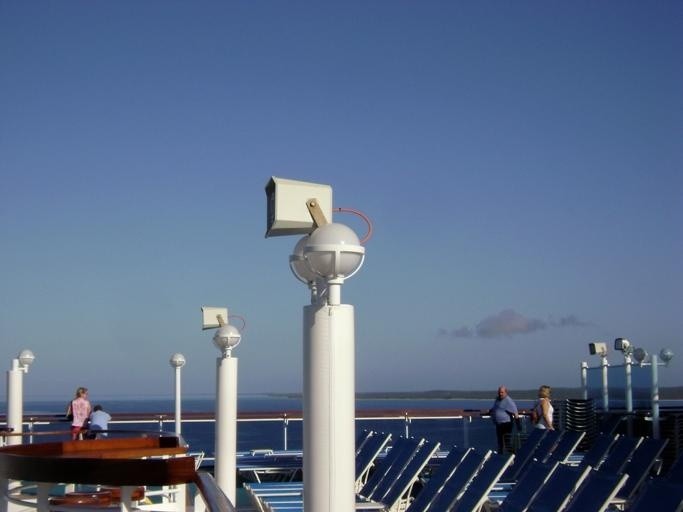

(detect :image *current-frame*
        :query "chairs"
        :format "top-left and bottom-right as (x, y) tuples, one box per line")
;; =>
(237, 427), (683, 512)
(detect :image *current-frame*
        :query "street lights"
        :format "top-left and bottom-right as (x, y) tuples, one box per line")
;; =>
(7, 349), (34, 445)
(212, 326), (245, 508)
(633, 348), (673, 439)
(170, 353), (186, 434)
(288, 224), (366, 512)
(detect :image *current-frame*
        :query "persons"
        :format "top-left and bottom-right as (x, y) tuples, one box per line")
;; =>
(533, 384), (556, 431)
(87, 405), (113, 439)
(65, 387), (90, 440)
(486, 385), (518, 450)
